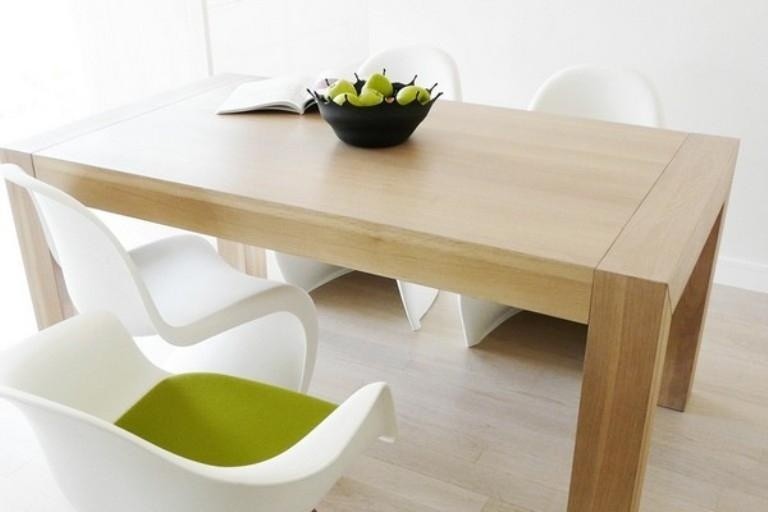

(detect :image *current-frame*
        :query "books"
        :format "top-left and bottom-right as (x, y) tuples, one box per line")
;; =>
(220, 64), (340, 114)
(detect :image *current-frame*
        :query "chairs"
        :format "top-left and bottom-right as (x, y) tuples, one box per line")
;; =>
(0, 307), (405, 511)
(266, 43), (458, 329)
(3, 162), (320, 392)
(455, 65), (666, 350)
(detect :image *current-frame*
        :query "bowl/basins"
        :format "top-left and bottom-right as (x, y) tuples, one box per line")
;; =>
(307, 69), (443, 147)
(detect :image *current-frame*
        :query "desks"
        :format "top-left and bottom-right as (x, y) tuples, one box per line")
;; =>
(0, 69), (743, 511)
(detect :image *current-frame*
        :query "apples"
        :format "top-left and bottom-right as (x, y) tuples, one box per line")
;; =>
(359, 88), (385, 106)
(362, 73), (394, 97)
(333, 92), (363, 107)
(324, 79), (357, 101)
(396, 85), (431, 106)
